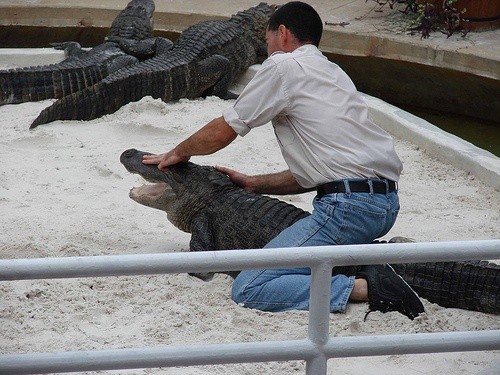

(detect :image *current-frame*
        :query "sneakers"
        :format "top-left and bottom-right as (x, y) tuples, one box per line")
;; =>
(364, 264), (425, 321)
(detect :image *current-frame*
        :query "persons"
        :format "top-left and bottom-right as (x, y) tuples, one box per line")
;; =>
(142, 1), (424, 320)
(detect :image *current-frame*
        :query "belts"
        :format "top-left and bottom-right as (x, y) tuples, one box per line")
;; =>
(317, 179), (396, 197)
